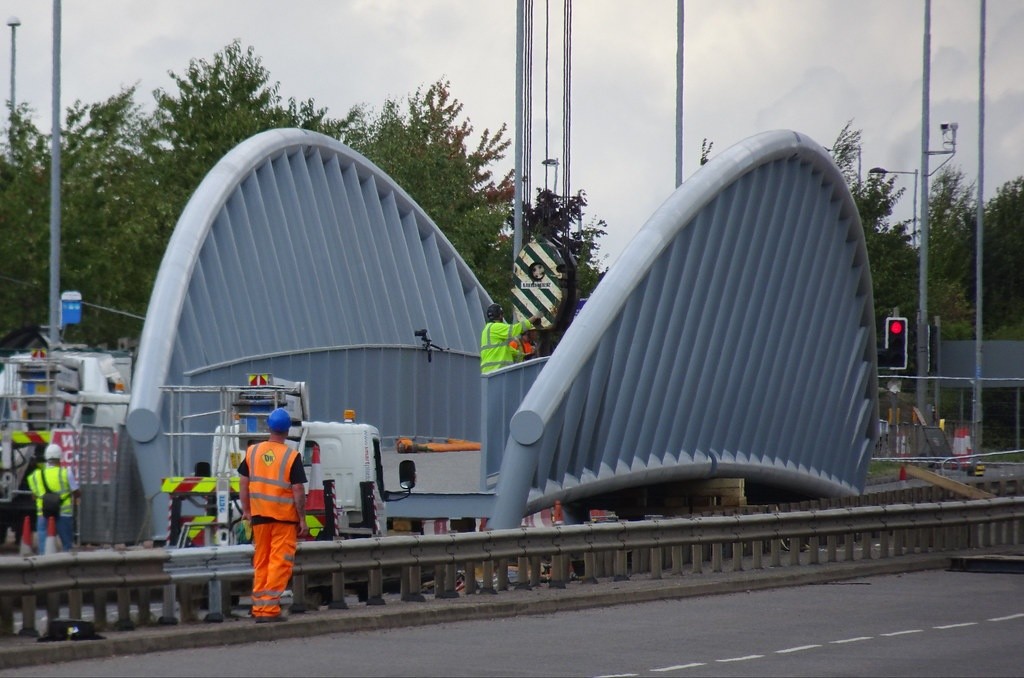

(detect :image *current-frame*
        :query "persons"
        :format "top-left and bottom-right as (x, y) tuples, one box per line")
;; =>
(236, 408), (307, 623)
(25, 443), (79, 555)
(479, 303), (544, 374)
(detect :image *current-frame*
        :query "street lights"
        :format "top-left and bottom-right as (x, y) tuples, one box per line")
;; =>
(869, 167), (919, 250)
(6, 16), (21, 112)
(541, 158), (559, 194)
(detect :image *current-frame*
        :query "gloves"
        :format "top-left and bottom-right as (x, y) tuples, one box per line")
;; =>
(534, 307), (548, 319)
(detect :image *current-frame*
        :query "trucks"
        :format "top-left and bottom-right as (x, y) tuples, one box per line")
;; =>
(158, 373), (417, 610)
(0, 350), (134, 547)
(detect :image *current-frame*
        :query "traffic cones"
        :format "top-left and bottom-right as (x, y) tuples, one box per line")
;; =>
(45, 517), (58, 556)
(896, 431), (911, 457)
(951, 427), (973, 469)
(19, 515), (33, 556)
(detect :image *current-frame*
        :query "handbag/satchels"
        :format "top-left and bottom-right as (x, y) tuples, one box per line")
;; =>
(43, 493), (60, 521)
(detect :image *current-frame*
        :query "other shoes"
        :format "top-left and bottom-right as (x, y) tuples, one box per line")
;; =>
(254, 611), (287, 622)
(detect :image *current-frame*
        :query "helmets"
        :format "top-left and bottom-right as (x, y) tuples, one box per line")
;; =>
(266, 409), (291, 432)
(487, 304), (504, 317)
(45, 444), (61, 460)
(520, 323), (536, 334)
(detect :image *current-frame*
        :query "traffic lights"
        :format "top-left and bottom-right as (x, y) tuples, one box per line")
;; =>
(886, 317), (907, 370)
